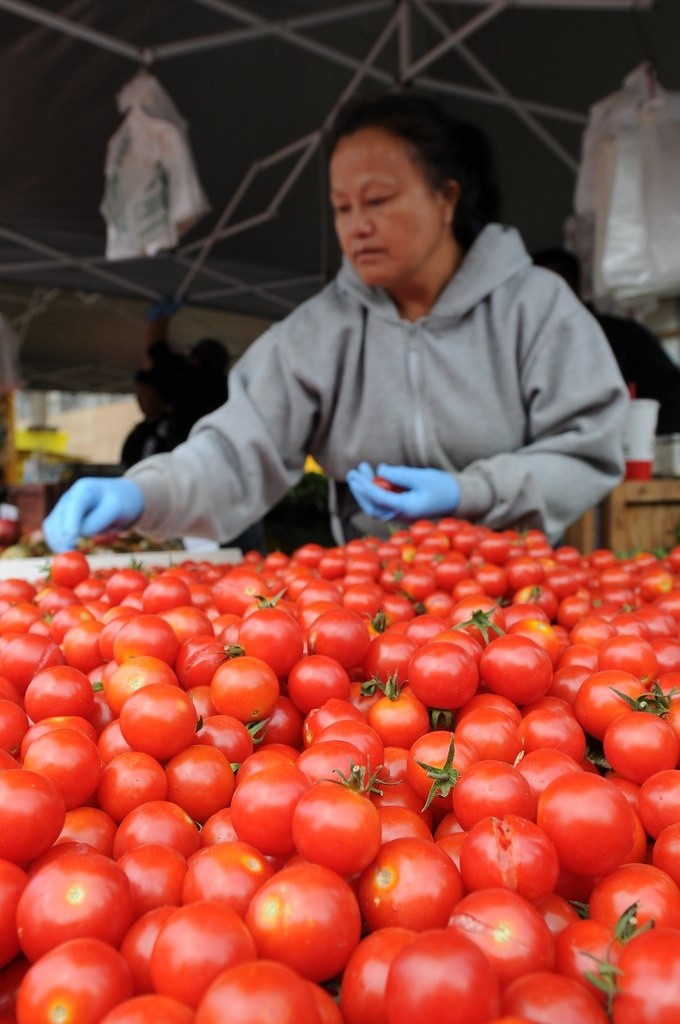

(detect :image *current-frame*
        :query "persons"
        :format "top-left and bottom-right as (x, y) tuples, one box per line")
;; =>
(532, 247), (679, 435)
(121, 284), (230, 470)
(41, 91), (630, 558)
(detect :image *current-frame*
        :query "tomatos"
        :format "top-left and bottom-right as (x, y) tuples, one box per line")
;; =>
(372, 477), (406, 492)
(0, 518), (680, 1024)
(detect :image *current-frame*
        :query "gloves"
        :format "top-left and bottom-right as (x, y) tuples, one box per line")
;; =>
(346, 462), (461, 522)
(42, 476), (144, 556)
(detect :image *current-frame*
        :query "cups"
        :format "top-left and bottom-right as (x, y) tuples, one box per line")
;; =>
(623, 399), (660, 481)
